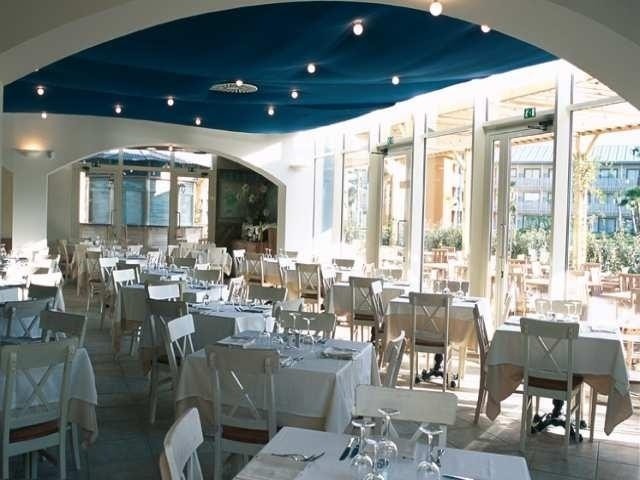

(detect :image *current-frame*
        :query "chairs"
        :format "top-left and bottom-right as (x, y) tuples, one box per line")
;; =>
(74, 235), (469, 479)
(1, 237), (89, 479)
(472, 235), (638, 479)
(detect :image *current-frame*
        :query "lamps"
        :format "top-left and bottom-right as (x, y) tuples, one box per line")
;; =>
(166, 95), (175, 107)
(307, 63), (316, 74)
(114, 104), (122, 114)
(236, 79), (244, 86)
(391, 75), (400, 85)
(429, 2), (442, 17)
(36, 85), (46, 96)
(196, 117), (202, 125)
(40, 111), (48, 120)
(352, 18), (364, 35)
(290, 89), (299, 99)
(480, 24), (491, 33)
(268, 105), (275, 116)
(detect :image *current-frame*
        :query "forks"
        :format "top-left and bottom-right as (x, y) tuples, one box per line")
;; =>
(270, 450), (325, 463)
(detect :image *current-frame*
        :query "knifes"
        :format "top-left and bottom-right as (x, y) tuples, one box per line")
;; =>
(338, 436), (360, 462)
(288, 356), (304, 368)
(442, 474), (473, 480)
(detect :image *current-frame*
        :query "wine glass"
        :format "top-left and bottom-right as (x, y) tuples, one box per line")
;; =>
(259, 311), (317, 353)
(202, 282), (247, 311)
(263, 248), (288, 259)
(146, 255), (177, 273)
(349, 406), (446, 480)
(433, 279), (470, 299)
(533, 297), (584, 325)
